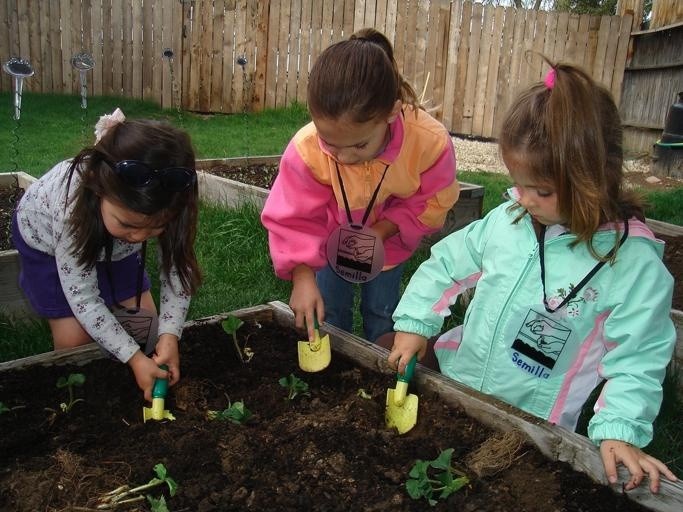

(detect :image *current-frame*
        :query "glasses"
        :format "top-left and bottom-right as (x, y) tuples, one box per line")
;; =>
(98, 151), (196, 192)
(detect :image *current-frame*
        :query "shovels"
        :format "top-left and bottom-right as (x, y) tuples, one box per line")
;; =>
(385, 352), (418, 436)
(298, 312), (331, 372)
(143, 353), (176, 424)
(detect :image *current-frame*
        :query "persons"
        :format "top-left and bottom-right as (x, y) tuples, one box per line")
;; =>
(260, 26), (462, 343)
(385, 48), (680, 496)
(9, 105), (206, 405)
